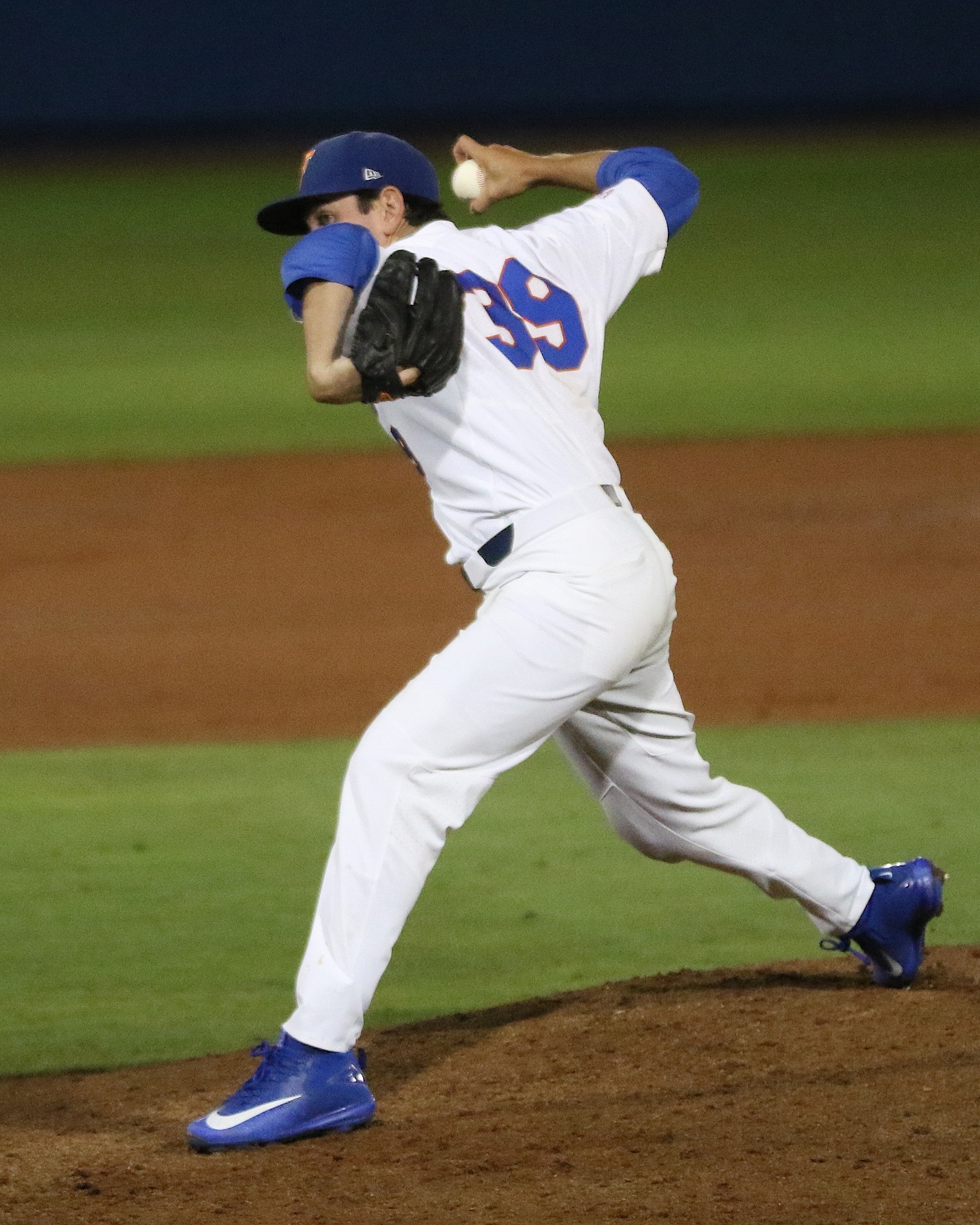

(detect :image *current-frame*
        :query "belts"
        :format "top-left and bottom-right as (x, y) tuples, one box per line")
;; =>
(462, 486), (633, 591)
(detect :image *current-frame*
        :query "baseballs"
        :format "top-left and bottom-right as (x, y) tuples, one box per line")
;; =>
(452, 158), (484, 201)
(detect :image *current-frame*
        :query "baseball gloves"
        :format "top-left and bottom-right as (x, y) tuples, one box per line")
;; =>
(349, 250), (464, 402)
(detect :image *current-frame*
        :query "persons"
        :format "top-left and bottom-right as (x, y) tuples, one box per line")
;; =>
(185, 130), (947, 1152)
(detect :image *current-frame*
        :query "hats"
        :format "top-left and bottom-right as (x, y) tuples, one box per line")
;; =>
(257, 132), (441, 235)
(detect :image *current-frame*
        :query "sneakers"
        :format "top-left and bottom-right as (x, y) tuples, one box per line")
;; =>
(820, 857), (944, 986)
(186, 1034), (376, 1145)
